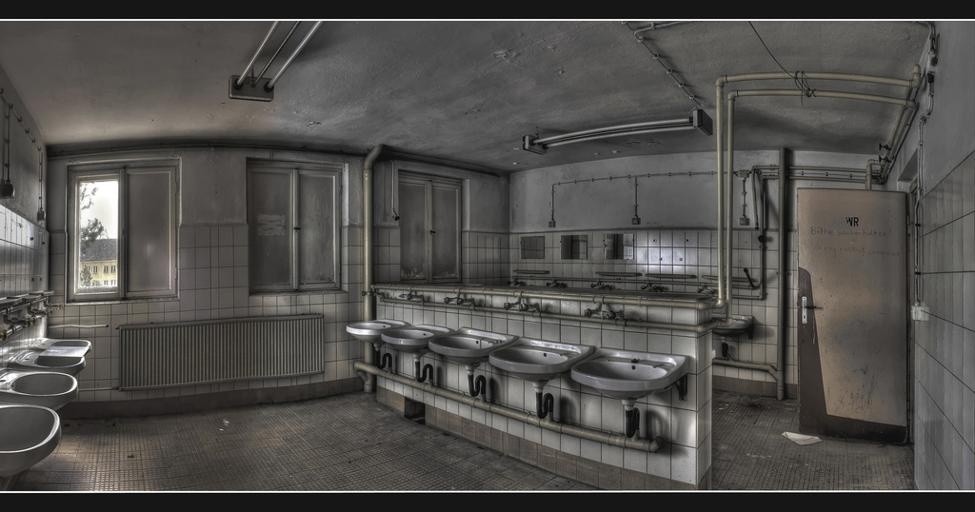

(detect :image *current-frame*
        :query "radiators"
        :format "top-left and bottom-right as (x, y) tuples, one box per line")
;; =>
(113, 310), (325, 391)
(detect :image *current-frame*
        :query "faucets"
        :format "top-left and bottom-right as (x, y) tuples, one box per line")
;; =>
(697, 282), (718, 294)
(505, 291), (539, 313)
(361, 286), (384, 301)
(507, 278), (527, 287)
(589, 279), (609, 291)
(1, 296), (63, 343)
(544, 279), (566, 288)
(397, 289), (424, 303)
(584, 296), (617, 320)
(440, 289), (477, 309)
(640, 279), (663, 292)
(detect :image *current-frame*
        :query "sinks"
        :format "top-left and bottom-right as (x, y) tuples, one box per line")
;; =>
(571, 348), (687, 407)
(380, 322), (453, 356)
(427, 326), (519, 369)
(712, 312), (754, 337)
(4, 349), (86, 375)
(488, 337), (595, 387)
(31, 337), (93, 354)
(345, 321), (408, 344)
(0, 402), (61, 478)
(0, 368), (78, 409)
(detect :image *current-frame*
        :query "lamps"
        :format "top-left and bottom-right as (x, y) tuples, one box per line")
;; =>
(521, 107), (710, 156)
(228, 21), (327, 102)
